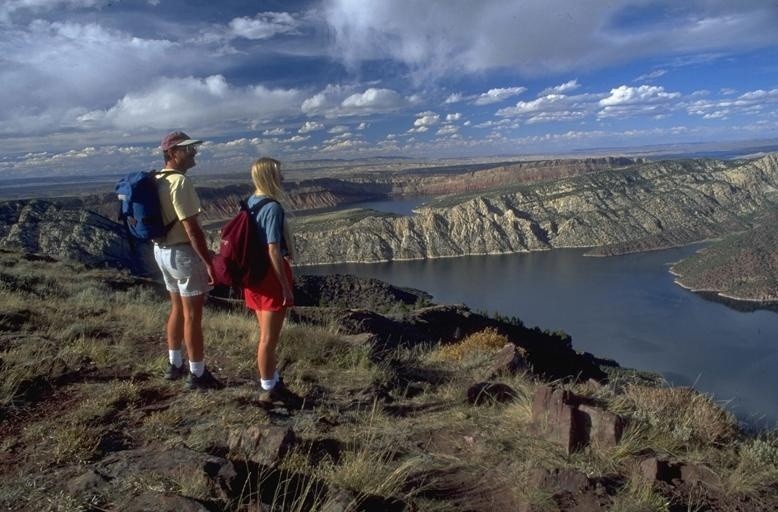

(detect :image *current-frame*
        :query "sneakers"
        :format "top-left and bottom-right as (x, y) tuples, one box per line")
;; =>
(186, 366), (226, 390)
(164, 361), (189, 380)
(259, 380), (304, 407)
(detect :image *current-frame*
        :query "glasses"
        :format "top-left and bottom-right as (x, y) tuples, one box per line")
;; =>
(182, 146), (197, 152)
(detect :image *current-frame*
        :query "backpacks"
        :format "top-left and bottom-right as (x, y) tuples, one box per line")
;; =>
(212, 195), (280, 286)
(115, 168), (181, 242)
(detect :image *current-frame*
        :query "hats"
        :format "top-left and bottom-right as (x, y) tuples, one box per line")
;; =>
(161, 130), (203, 153)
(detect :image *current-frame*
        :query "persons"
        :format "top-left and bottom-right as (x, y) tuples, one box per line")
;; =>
(150, 130), (226, 390)
(241, 158), (299, 407)
